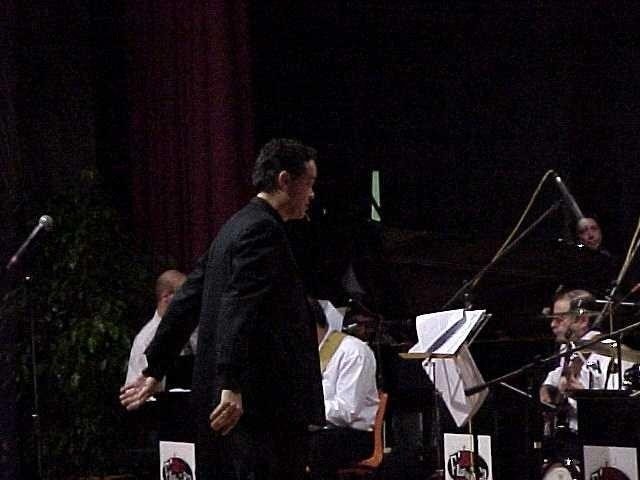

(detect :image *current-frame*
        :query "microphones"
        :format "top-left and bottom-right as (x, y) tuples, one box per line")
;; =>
(555, 175), (591, 232)
(5, 215), (55, 272)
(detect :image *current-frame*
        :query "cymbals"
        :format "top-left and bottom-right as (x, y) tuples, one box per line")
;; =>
(574, 338), (640, 364)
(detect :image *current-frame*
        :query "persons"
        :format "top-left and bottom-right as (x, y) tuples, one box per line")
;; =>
(535, 289), (639, 435)
(109, 138), (327, 476)
(563, 216), (638, 300)
(300, 297), (381, 477)
(121, 268), (202, 429)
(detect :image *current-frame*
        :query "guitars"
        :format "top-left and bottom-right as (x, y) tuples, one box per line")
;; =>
(543, 339), (597, 442)
